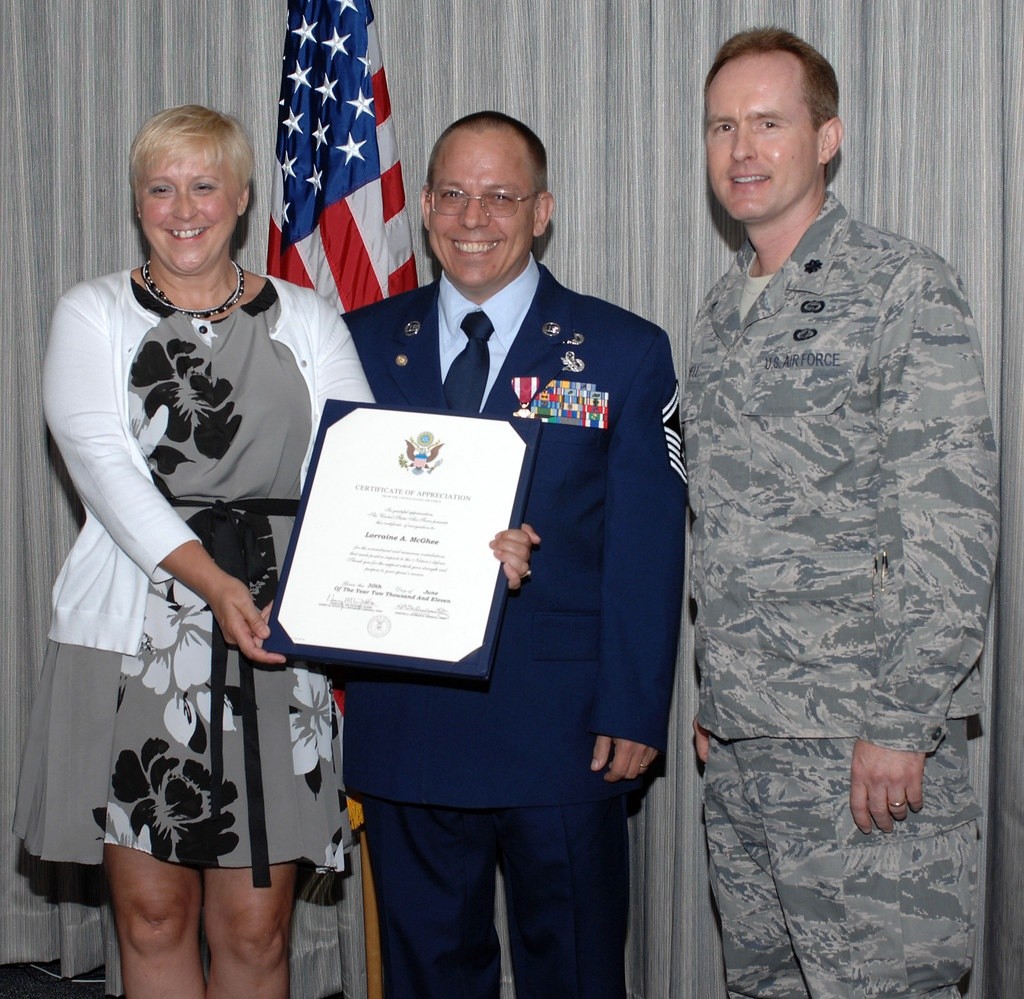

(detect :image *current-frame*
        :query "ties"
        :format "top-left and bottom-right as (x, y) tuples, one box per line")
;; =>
(443, 310), (495, 414)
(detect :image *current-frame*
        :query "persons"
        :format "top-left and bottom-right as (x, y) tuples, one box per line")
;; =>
(13, 104), (541, 997)
(682, 24), (1002, 999)
(339, 110), (689, 999)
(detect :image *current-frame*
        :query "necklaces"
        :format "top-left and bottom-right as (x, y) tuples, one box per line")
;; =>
(141, 257), (245, 318)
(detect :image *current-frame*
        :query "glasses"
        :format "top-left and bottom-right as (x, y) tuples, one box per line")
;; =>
(427, 192), (539, 218)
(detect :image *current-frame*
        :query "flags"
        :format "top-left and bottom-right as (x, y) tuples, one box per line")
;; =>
(266, 0), (418, 832)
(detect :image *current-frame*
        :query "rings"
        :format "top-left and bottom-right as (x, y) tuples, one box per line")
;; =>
(640, 764), (648, 768)
(520, 569), (532, 579)
(890, 801), (905, 807)
